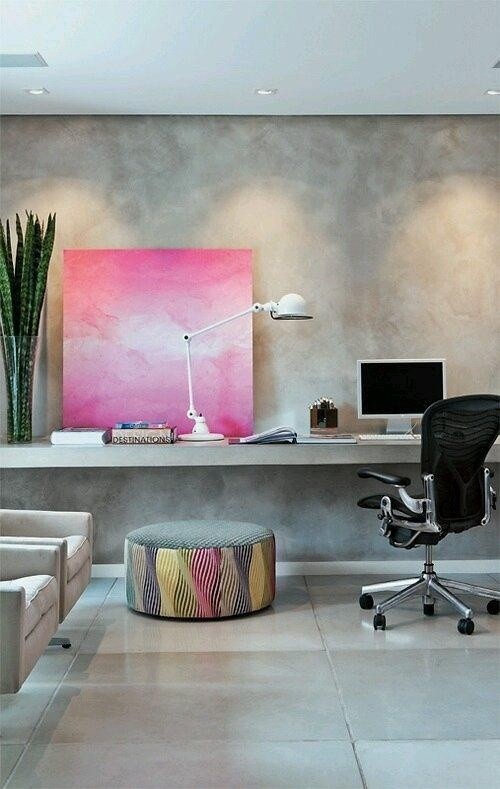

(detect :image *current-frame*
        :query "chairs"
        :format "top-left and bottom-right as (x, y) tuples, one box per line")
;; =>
(356, 395), (500, 635)
(1, 544), (71, 694)
(0, 507), (94, 621)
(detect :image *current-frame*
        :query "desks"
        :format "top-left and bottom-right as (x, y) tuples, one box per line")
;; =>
(0, 444), (500, 468)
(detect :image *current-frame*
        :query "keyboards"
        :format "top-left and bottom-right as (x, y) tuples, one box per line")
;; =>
(359, 434), (422, 441)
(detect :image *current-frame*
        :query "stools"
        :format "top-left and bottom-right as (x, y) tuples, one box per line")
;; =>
(125, 520), (276, 619)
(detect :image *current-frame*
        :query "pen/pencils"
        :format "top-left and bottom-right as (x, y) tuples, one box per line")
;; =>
(312, 397), (334, 409)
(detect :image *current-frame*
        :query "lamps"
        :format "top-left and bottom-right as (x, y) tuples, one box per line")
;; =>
(177, 292), (313, 441)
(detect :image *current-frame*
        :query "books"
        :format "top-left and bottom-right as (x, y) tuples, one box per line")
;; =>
(230, 426), (356, 444)
(50, 419), (178, 445)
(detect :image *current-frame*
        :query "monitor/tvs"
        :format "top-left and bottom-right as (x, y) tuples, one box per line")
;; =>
(357, 358), (447, 434)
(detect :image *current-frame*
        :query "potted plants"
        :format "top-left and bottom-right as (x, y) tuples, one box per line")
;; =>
(0, 209), (57, 444)
